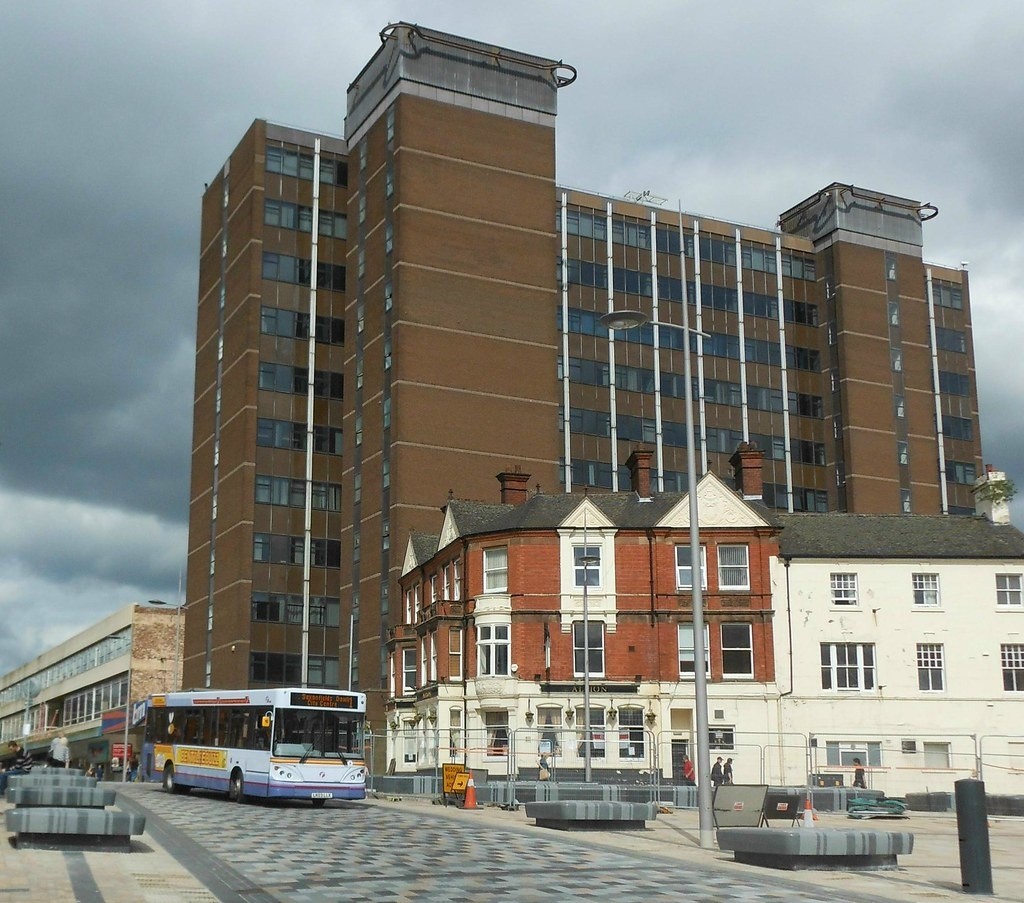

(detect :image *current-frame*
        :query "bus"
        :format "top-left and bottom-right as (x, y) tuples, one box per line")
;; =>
(140, 687), (372, 806)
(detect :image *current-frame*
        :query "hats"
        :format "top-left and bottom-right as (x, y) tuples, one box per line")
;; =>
(682, 755), (688, 759)
(717, 757), (723, 760)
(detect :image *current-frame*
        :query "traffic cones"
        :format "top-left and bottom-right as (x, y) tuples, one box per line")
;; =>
(461, 769), (484, 809)
(800, 797), (816, 826)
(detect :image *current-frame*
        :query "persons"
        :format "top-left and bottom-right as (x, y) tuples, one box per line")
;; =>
(538, 753), (551, 782)
(0, 741), (33, 796)
(852, 757), (866, 789)
(683, 755), (694, 786)
(87, 753), (141, 782)
(712, 756), (724, 785)
(723, 758), (736, 784)
(50, 730), (70, 769)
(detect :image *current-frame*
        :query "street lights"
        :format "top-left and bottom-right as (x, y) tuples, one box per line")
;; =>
(149, 600), (181, 693)
(600, 309), (716, 850)
(109, 635), (133, 782)
